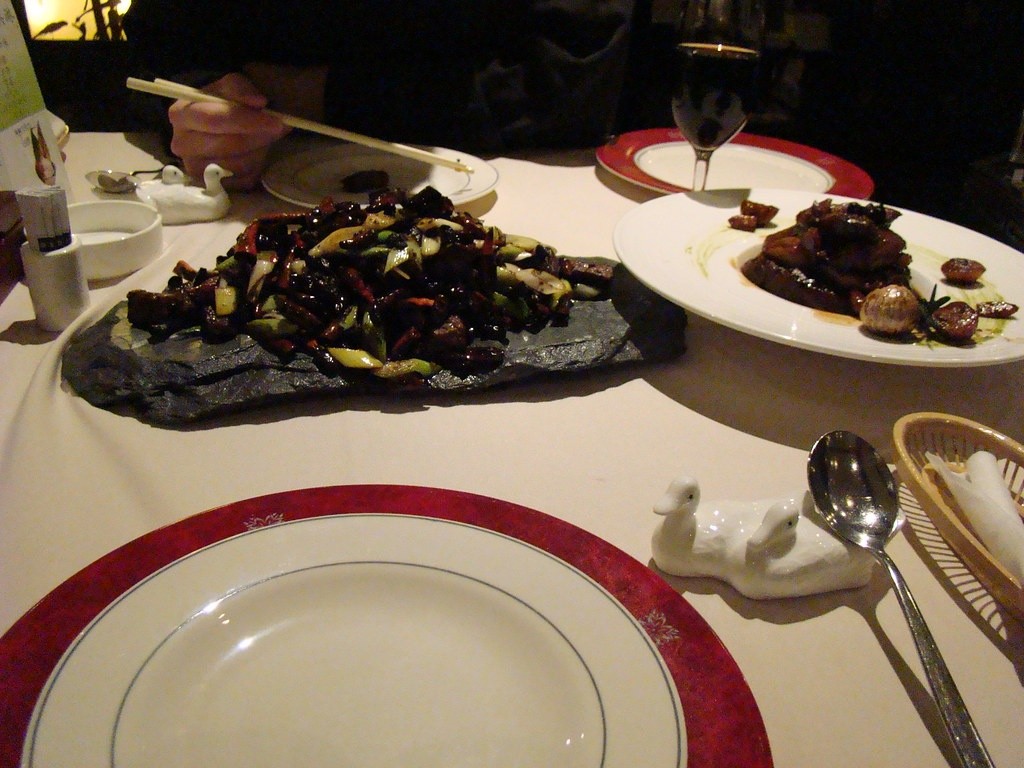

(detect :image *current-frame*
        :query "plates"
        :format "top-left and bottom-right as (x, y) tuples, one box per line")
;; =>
(1, 485), (774, 768)
(597, 126), (874, 201)
(260, 139), (500, 209)
(612, 187), (1024, 367)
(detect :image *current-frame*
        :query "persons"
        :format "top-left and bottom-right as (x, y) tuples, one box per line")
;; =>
(121, 0), (637, 196)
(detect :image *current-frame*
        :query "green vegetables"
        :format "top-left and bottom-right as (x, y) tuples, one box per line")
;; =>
(916, 283), (952, 333)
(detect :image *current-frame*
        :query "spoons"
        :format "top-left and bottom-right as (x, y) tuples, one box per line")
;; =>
(808, 429), (996, 768)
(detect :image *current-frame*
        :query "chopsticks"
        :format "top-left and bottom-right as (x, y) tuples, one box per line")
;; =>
(122, 78), (475, 175)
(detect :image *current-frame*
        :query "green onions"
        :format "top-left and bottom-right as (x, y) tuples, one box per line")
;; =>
(216, 210), (600, 377)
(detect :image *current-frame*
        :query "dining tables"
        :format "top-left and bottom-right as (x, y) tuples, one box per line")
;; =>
(0, 133), (1023, 768)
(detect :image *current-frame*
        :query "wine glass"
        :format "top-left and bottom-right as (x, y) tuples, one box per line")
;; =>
(668, 1), (768, 191)
